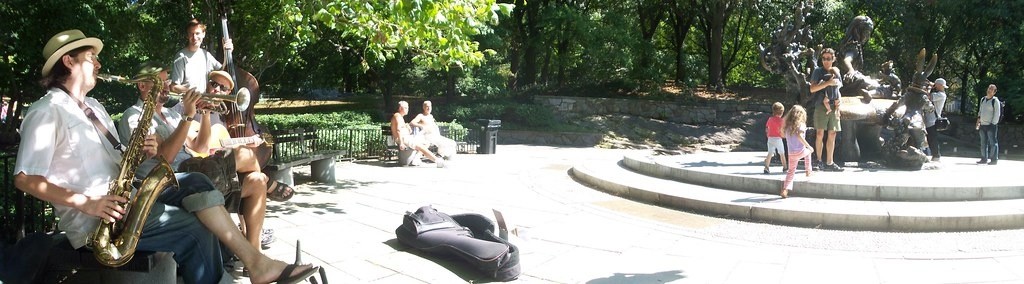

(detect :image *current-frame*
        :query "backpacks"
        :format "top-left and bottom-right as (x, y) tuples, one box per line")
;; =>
(982, 96), (1004, 123)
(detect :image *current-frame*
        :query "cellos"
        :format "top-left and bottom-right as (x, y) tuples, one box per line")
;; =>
(214, 0), (274, 171)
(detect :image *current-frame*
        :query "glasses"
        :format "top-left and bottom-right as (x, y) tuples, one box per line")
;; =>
(77, 53), (99, 62)
(822, 57), (833, 61)
(211, 80), (230, 92)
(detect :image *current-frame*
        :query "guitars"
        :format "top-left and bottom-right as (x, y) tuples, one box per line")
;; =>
(185, 119), (264, 158)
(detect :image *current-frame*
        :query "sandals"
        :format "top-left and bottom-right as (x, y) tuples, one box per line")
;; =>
(277, 263), (319, 284)
(266, 178), (294, 202)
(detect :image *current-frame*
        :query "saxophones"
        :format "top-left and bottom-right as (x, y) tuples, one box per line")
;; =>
(84, 70), (180, 269)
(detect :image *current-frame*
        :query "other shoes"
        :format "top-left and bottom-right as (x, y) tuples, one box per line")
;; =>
(816, 162), (829, 171)
(764, 168), (771, 175)
(238, 224), (274, 235)
(826, 162), (844, 172)
(976, 160), (987, 164)
(783, 168), (788, 171)
(243, 266), (250, 276)
(260, 234), (276, 249)
(807, 172), (812, 176)
(781, 191), (789, 198)
(988, 162), (997, 165)
(931, 156), (938, 161)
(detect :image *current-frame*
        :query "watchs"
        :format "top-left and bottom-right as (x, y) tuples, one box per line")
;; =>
(181, 113), (193, 122)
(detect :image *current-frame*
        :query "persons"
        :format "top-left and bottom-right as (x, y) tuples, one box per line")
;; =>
(924, 78), (949, 162)
(169, 14), (276, 247)
(779, 104), (814, 198)
(975, 84), (1002, 166)
(14, 29), (321, 284)
(390, 101), (453, 168)
(836, 15), (886, 101)
(115, 60), (293, 277)
(410, 100), (458, 160)
(821, 73), (842, 118)
(811, 48), (846, 172)
(0, 95), (17, 124)
(762, 102), (789, 174)
(876, 60), (901, 95)
(877, 70), (935, 170)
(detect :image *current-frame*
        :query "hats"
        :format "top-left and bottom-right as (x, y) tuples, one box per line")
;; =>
(42, 29), (104, 78)
(935, 78), (950, 89)
(209, 71), (234, 90)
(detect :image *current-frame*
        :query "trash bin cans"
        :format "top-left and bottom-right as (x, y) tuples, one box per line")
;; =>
(474, 118), (501, 154)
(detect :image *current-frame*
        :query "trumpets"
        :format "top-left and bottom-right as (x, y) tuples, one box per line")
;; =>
(164, 87), (251, 116)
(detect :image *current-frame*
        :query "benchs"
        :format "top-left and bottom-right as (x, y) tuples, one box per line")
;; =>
(42, 231), (177, 284)
(262, 126), (348, 188)
(380, 123), (466, 166)
